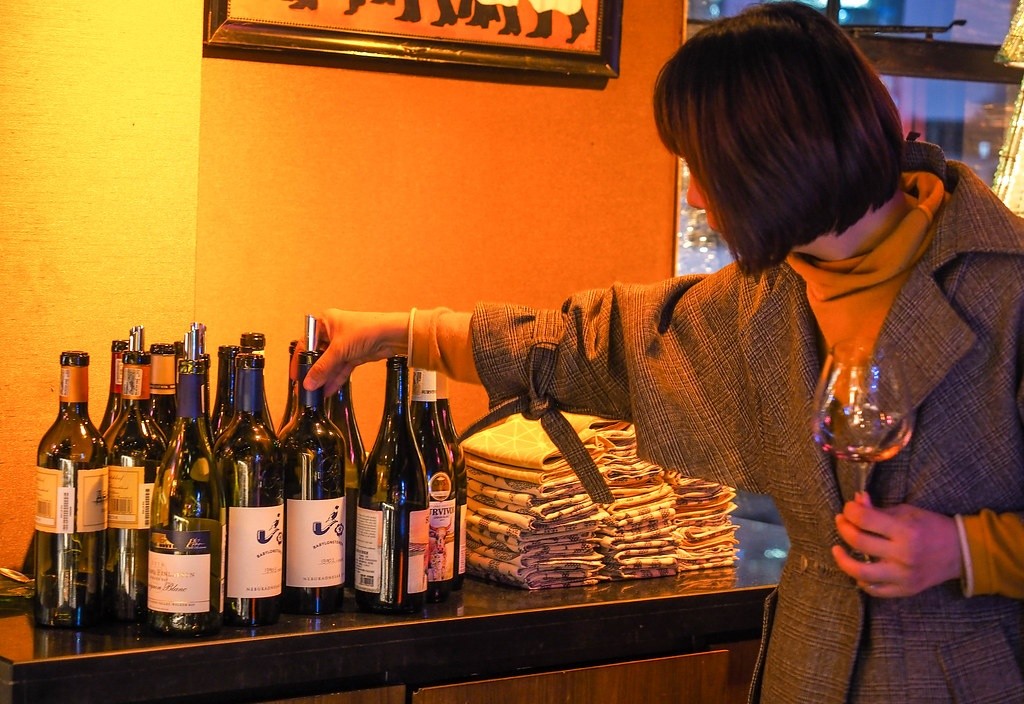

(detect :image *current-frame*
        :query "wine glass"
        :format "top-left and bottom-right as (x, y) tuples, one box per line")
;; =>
(812, 338), (915, 589)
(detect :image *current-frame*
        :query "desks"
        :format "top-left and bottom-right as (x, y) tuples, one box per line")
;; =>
(0, 516), (790, 704)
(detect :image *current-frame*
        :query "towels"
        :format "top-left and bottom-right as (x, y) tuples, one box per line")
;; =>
(461, 404), (738, 593)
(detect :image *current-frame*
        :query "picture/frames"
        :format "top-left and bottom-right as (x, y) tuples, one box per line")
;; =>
(204, 0), (623, 79)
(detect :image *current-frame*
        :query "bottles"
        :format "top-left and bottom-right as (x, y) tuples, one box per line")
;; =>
(34, 314), (467, 639)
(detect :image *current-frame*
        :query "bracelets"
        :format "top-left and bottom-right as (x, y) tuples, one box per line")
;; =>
(407, 307), (416, 367)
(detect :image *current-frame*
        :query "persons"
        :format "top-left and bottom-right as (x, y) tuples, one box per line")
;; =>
(288, 0), (1024, 703)
